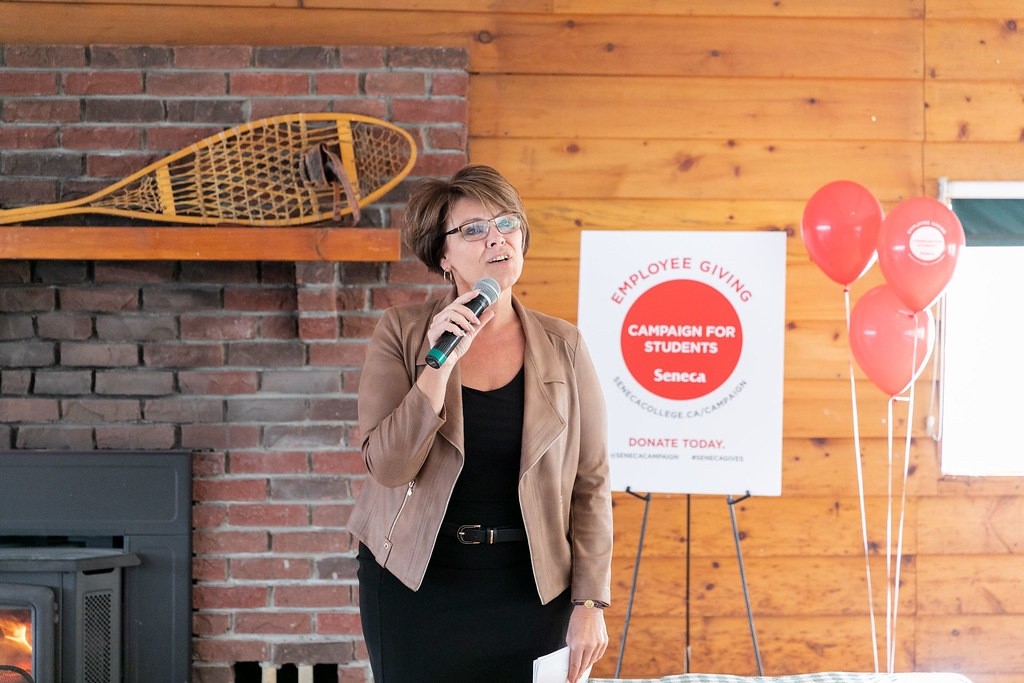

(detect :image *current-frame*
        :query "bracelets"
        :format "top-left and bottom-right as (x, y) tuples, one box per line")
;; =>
(572, 599), (604, 609)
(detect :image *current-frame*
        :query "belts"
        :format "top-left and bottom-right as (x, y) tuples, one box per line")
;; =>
(439, 522), (525, 544)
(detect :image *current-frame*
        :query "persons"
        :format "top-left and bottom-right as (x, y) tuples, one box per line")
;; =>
(346, 163), (615, 683)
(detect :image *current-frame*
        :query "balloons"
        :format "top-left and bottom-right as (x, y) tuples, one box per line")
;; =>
(876, 195), (965, 321)
(845, 283), (937, 408)
(800, 181), (889, 292)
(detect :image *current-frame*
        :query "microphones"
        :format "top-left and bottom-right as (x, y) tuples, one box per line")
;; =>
(425, 277), (501, 369)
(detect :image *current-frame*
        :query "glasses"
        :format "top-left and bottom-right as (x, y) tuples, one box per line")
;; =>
(445, 212), (521, 242)
